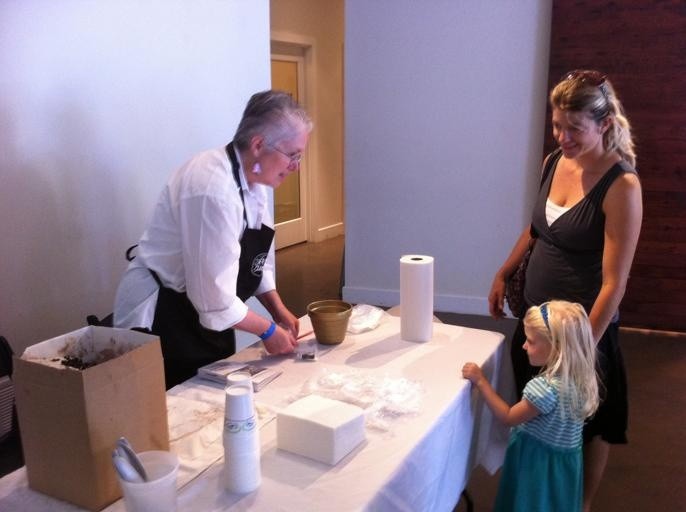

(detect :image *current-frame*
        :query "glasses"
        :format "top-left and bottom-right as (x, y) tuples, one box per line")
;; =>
(271, 143), (304, 163)
(561, 70), (608, 98)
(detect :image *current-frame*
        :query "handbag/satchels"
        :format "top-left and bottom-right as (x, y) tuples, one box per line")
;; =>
(505, 252), (529, 317)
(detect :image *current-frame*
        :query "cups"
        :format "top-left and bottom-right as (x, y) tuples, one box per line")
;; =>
(218, 366), (264, 498)
(118, 449), (180, 511)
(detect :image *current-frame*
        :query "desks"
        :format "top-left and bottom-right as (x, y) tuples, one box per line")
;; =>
(0, 304), (506, 512)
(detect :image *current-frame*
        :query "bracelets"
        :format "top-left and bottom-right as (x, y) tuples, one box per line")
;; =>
(260, 322), (276, 340)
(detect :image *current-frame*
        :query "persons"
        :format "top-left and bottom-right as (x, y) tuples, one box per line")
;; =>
(491, 69), (646, 512)
(113, 90), (316, 390)
(461, 297), (610, 512)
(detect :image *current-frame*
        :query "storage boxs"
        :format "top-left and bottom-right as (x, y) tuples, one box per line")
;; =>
(11, 323), (172, 512)
